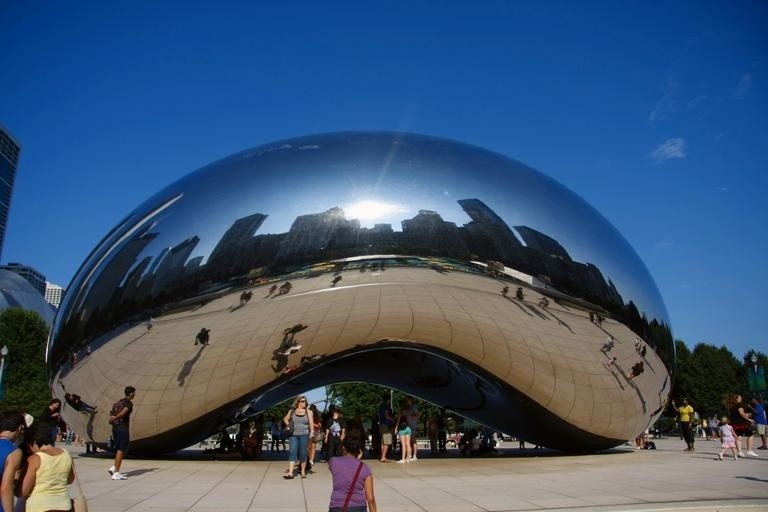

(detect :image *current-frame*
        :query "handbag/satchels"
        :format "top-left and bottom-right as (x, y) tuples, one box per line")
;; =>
(281, 425), (293, 439)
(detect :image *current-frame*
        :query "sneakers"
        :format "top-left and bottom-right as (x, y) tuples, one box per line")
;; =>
(283, 475), (307, 479)
(380, 456), (419, 463)
(718, 445), (768, 461)
(109, 465), (129, 480)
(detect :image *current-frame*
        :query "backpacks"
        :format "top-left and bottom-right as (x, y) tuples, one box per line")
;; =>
(109, 402), (124, 426)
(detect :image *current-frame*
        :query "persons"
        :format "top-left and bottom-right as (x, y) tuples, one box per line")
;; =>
(239, 281), (293, 303)
(14, 392), (98, 483)
(330, 274), (343, 287)
(271, 418), (287, 451)
(371, 396), (421, 463)
(194, 328), (212, 346)
(145, 316), (154, 331)
(501, 284), (649, 381)
(1, 407), (27, 511)
(105, 385), (135, 481)
(324, 435), (378, 512)
(284, 323), (307, 334)
(14, 419), (79, 512)
(276, 344), (301, 355)
(309, 404), (367, 464)
(279, 395), (316, 479)
(634, 392), (768, 462)
(427, 408), (525, 454)
(219, 414), (264, 457)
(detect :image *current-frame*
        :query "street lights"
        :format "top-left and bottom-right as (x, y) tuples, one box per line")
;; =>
(1, 346), (10, 383)
(746, 353), (767, 400)
